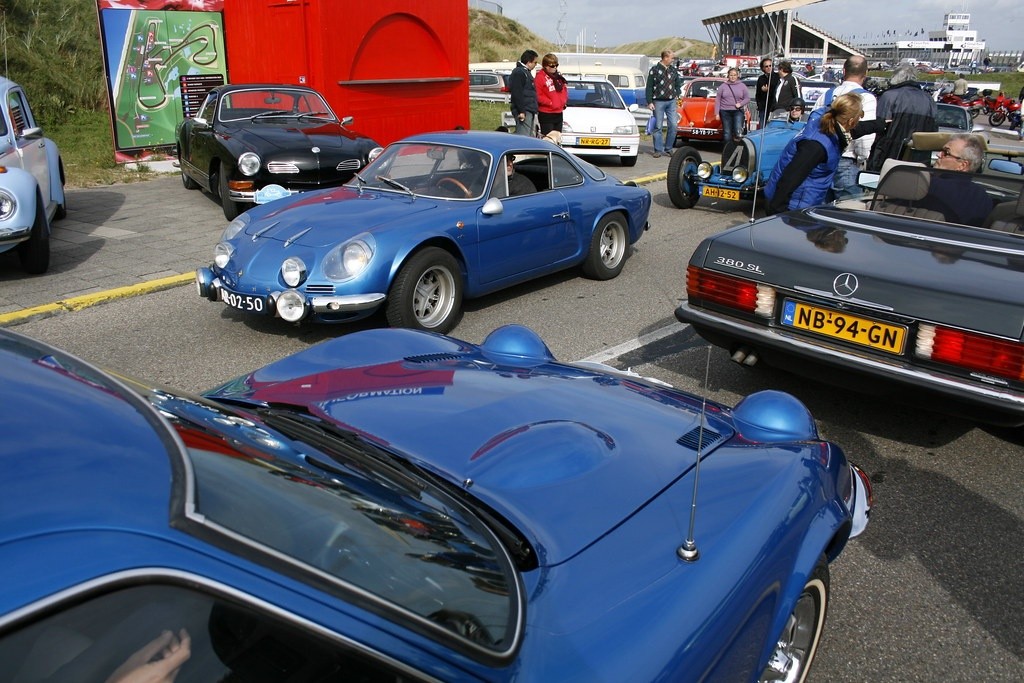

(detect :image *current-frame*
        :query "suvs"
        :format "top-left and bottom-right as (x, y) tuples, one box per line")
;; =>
(469, 71), (510, 93)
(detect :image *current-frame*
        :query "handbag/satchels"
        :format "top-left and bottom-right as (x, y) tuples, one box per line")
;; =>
(745, 110), (751, 124)
(645, 110), (656, 135)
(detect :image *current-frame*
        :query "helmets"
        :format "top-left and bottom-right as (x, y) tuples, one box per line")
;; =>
(789, 98), (806, 108)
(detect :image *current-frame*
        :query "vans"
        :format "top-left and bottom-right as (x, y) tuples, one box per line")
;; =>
(469, 59), (543, 78)
(556, 67), (647, 107)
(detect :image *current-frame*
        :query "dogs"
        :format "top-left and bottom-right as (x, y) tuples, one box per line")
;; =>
(512, 130), (562, 162)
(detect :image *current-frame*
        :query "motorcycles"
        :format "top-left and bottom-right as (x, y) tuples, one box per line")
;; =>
(941, 89), (1023, 131)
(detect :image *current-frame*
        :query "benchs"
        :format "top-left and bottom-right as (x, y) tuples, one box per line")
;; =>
(515, 165), (573, 192)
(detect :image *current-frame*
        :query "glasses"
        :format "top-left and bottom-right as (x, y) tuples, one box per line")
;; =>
(941, 147), (963, 162)
(531, 61), (538, 64)
(855, 111), (864, 119)
(547, 64), (559, 68)
(763, 65), (771, 68)
(790, 107), (802, 112)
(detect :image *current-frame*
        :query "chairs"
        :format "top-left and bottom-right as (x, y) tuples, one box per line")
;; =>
(696, 90), (708, 99)
(867, 158), (945, 222)
(990, 183), (1024, 235)
(584, 93), (602, 103)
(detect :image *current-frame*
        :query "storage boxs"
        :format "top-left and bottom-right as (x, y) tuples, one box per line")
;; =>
(983, 89), (993, 96)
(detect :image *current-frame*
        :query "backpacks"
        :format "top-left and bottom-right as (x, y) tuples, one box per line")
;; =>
(807, 87), (870, 123)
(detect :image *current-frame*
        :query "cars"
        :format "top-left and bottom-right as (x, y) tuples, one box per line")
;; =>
(674, 131), (1024, 412)
(791, 65), (843, 81)
(868, 62), (890, 71)
(0, 323), (873, 683)
(943, 67), (980, 74)
(0, 77), (66, 278)
(175, 83), (390, 222)
(196, 130), (652, 332)
(533, 76), (641, 167)
(915, 64), (943, 74)
(667, 108), (810, 209)
(673, 77), (751, 147)
(930, 102), (985, 160)
(677, 62), (763, 86)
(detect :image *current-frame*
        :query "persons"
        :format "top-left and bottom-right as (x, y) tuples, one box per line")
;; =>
(714, 68), (750, 143)
(953, 73), (968, 98)
(508, 49), (539, 137)
(983, 56), (992, 69)
(646, 50), (682, 158)
(103, 627), (192, 683)
(692, 61), (697, 72)
(714, 63), (720, 71)
(866, 61), (939, 172)
(911, 136), (993, 227)
(774, 60), (803, 110)
(755, 58), (781, 130)
(1018, 99), (1024, 142)
(812, 55), (877, 196)
(534, 54), (568, 136)
(470, 154), (537, 196)
(970, 58), (978, 74)
(788, 97), (806, 123)
(764, 93), (893, 216)
(822, 66), (834, 82)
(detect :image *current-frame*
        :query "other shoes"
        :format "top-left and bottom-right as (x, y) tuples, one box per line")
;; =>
(653, 151), (662, 158)
(666, 149), (673, 156)
